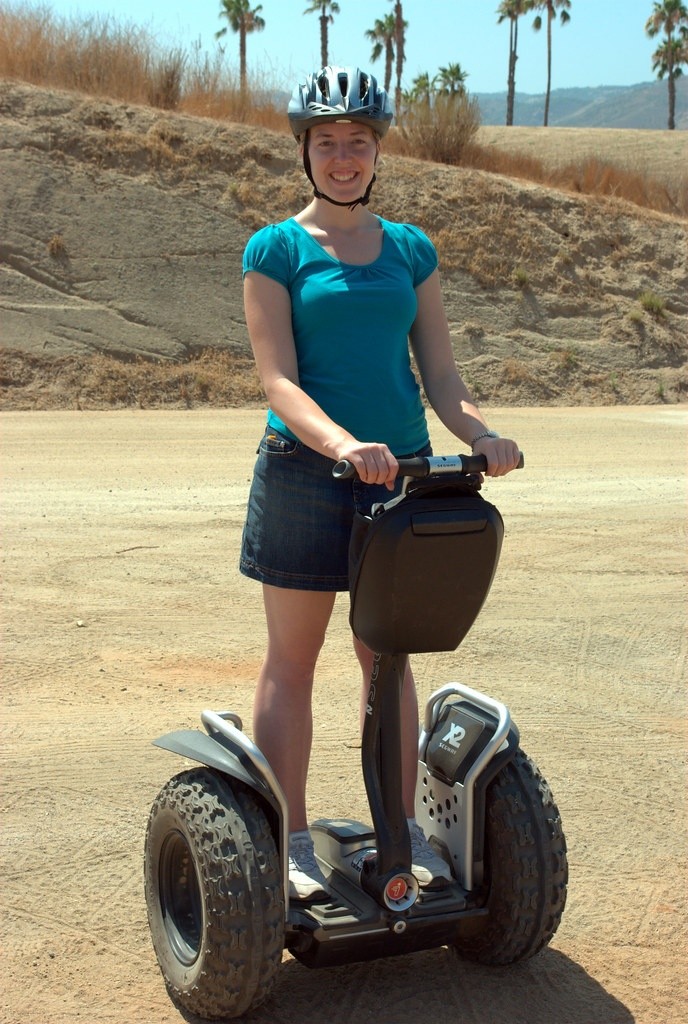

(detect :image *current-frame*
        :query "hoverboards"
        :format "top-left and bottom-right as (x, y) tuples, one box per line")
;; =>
(141, 452), (571, 1024)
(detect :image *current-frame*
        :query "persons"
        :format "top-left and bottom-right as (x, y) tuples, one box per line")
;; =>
(239, 67), (519, 907)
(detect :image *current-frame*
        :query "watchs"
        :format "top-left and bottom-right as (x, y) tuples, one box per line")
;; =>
(472, 431), (499, 451)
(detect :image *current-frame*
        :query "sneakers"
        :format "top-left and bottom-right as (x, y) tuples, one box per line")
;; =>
(289, 835), (331, 902)
(410, 827), (453, 888)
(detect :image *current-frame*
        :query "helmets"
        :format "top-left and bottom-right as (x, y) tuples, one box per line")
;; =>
(287, 64), (393, 143)
